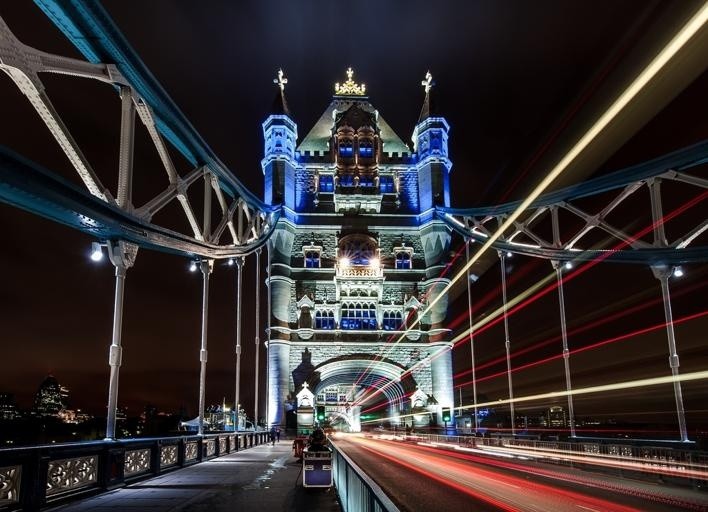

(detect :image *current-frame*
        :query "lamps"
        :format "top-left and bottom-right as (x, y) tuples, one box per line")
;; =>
(91, 243), (108, 262)
(506, 252), (512, 257)
(674, 266), (684, 277)
(561, 261), (572, 273)
(189, 260), (200, 273)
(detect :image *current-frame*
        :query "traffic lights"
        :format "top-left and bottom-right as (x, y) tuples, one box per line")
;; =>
(317, 405), (325, 420)
(443, 407), (451, 422)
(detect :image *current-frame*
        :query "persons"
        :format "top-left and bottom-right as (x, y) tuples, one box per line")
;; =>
(270, 426), (282, 446)
